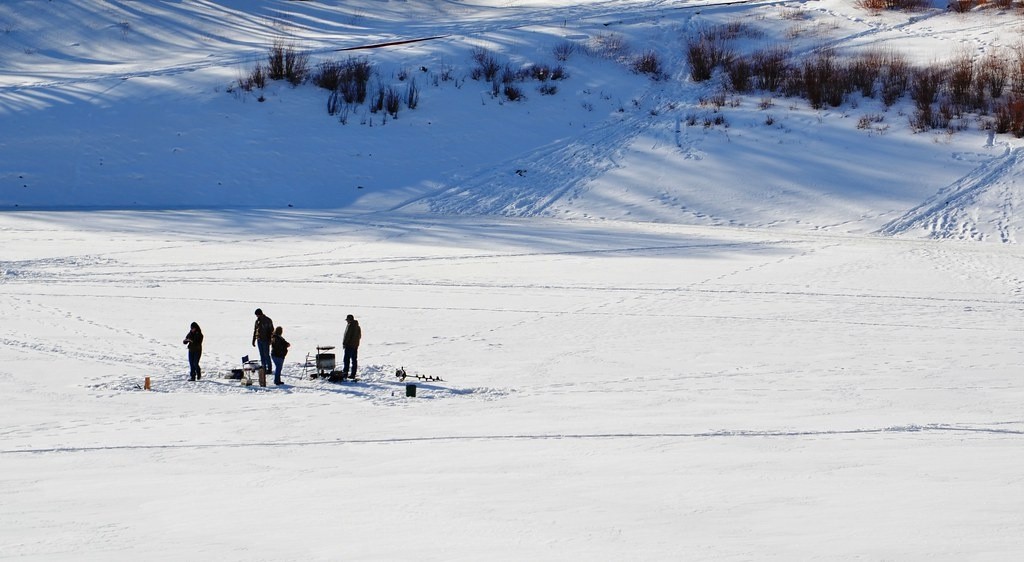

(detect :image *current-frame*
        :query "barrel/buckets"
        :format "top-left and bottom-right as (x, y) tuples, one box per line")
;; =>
(406, 384), (416, 398)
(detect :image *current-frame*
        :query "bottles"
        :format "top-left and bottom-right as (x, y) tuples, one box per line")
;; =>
(145, 377), (150, 391)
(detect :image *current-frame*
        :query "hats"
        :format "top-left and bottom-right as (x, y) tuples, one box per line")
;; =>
(255, 308), (262, 314)
(345, 315), (353, 320)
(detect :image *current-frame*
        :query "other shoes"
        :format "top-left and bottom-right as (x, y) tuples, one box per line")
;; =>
(348, 374), (355, 379)
(187, 376), (195, 381)
(197, 373), (201, 380)
(276, 381), (284, 385)
(265, 370), (272, 374)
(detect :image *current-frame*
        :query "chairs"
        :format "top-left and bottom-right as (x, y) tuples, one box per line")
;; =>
(241, 354), (263, 384)
(297, 352), (320, 381)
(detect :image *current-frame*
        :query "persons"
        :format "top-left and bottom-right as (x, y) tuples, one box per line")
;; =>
(343, 315), (361, 379)
(271, 327), (290, 385)
(252, 308), (274, 374)
(183, 322), (203, 381)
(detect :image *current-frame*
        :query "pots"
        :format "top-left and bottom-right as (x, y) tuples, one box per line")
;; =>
(315, 353), (335, 370)
